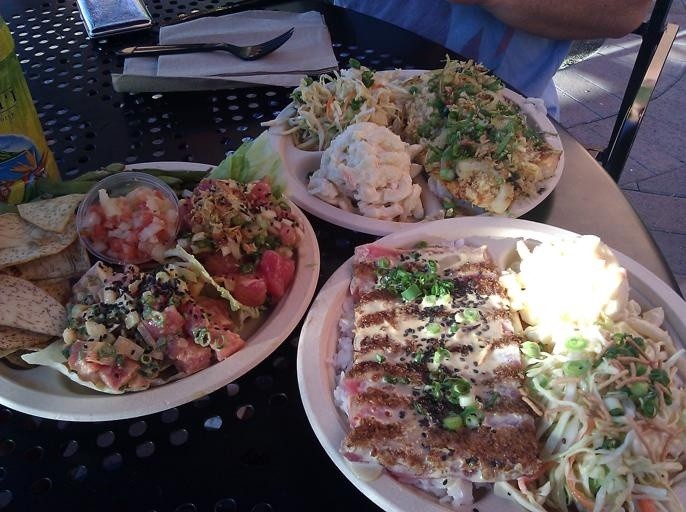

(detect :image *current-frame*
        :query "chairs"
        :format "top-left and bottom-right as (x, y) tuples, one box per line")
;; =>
(586, 0), (680, 183)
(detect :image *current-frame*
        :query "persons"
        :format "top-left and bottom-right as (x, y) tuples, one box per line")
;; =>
(329, 1), (658, 125)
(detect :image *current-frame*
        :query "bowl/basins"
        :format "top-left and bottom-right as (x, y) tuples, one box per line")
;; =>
(76, 172), (182, 268)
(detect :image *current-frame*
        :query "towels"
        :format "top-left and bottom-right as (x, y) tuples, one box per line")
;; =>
(158, 10), (339, 77)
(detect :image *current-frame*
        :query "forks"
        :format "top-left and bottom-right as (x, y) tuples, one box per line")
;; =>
(111, 27), (296, 64)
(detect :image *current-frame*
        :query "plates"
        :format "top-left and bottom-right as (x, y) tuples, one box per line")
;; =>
(1, 161), (322, 424)
(263, 66), (567, 237)
(295, 217), (686, 511)
